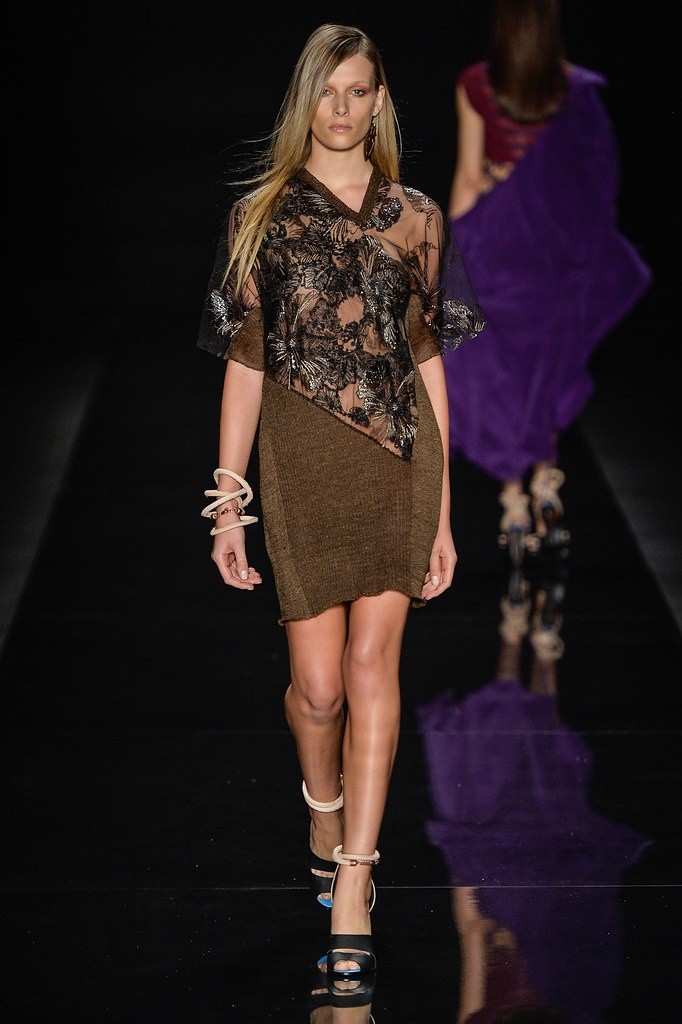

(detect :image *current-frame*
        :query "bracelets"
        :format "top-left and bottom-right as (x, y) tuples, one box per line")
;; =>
(200, 467), (259, 537)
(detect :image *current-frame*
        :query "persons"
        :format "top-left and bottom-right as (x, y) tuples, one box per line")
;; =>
(186, 25), (485, 981)
(310, 982), (373, 1024)
(419, 570), (648, 1024)
(440, 1), (647, 569)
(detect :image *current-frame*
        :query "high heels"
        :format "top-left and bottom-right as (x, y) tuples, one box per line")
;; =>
(302, 774), (343, 910)
(328, 844), (380, 977)
(531, 468), (565, 536)
(499, 493), (530, 562)
